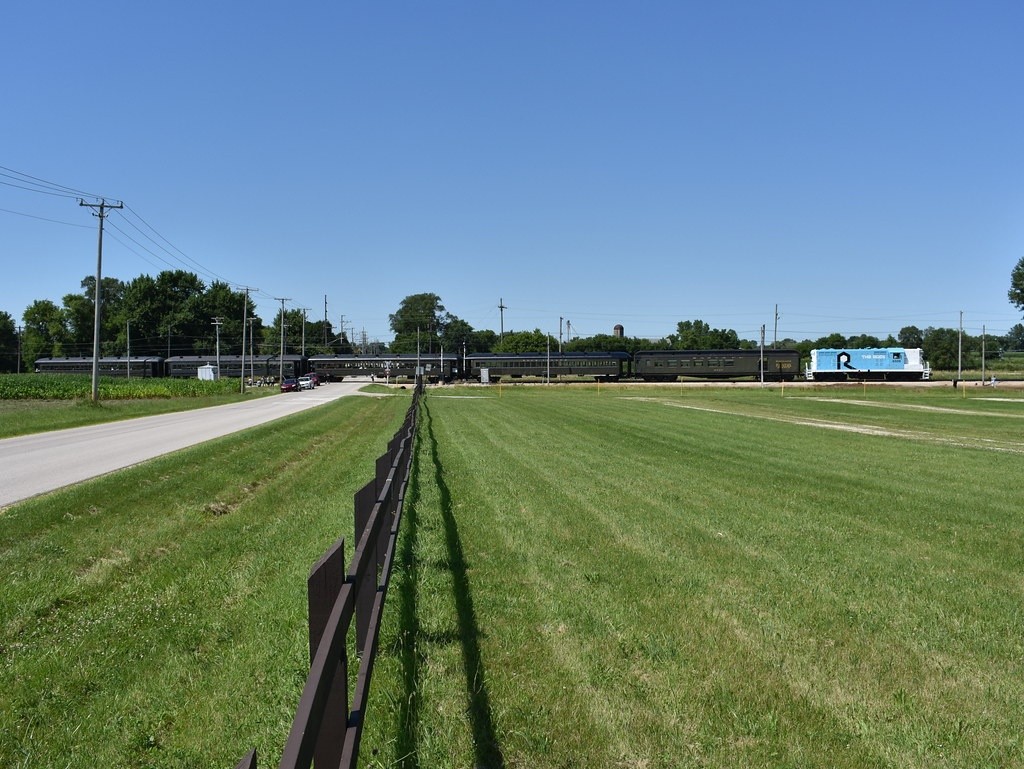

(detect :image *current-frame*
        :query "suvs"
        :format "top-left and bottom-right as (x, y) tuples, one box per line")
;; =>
(304, 373), (320, 385)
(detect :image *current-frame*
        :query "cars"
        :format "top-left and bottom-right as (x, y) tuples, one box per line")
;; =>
(281, 378), (301, 392)
(298, 377), (315, 389)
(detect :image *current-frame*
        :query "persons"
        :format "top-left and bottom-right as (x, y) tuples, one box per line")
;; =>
(370, 372), (374, 382)
(270, 376), (274, 387)
(323, 373), (330, 384)
(257, 379), (261, 387)
(267, 376), (270, 387)
(262, 376), (266, 387)
(248, 378), (253, 385)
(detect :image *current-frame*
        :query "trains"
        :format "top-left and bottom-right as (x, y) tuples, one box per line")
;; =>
(33, 348), (931, 383)
